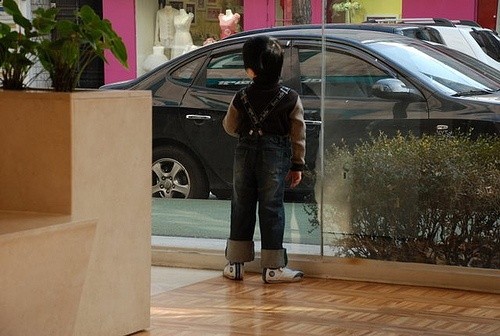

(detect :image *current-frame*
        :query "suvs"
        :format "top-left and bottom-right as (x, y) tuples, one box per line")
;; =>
(222, 17), (496, 63)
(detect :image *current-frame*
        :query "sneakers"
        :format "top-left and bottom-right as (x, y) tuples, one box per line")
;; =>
(262, 266), (304, 284)
(223, 262), (245, 280)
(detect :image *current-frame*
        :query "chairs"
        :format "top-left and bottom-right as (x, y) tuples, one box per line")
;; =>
(303, 63), (373, 97)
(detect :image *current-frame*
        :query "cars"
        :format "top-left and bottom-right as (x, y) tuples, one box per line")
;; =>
(97, 30), (495, 198)
